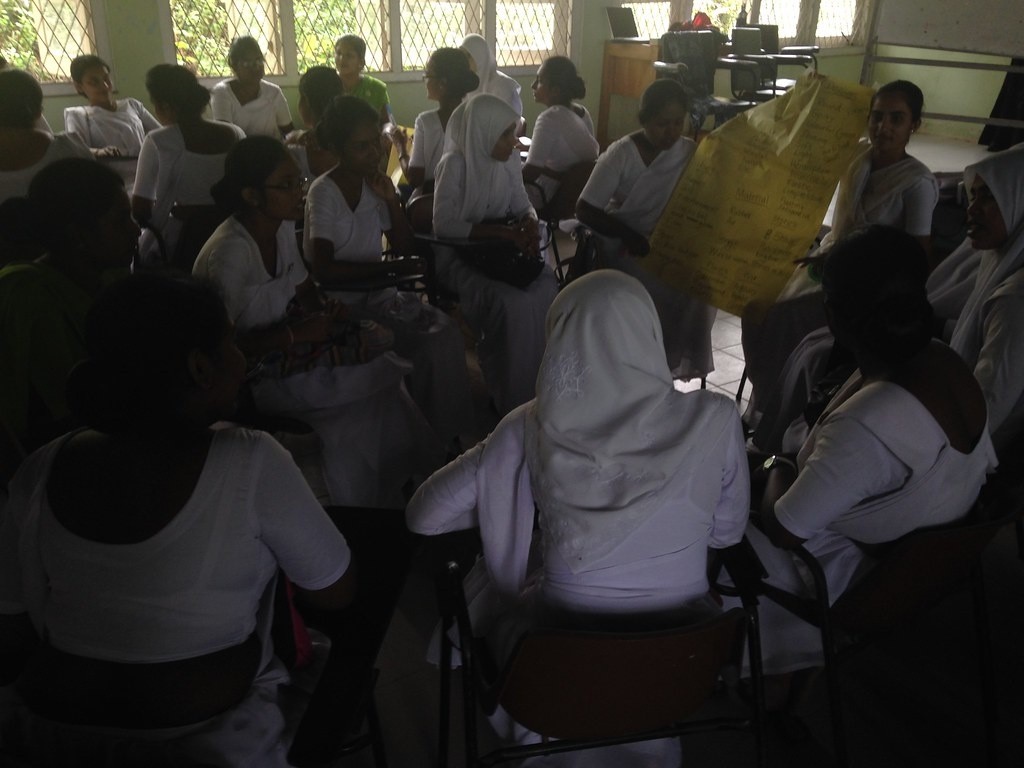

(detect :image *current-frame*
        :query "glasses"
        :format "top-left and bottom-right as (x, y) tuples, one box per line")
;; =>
(262, 177), (307, 193)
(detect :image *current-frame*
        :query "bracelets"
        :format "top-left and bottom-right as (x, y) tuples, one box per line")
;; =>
(399, 153), (409, 160)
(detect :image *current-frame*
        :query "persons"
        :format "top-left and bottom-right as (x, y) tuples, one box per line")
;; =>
(511, 56), (600, 223)
(333, 35), (392, 172)
(745, 228), (1000, 674)
(63, 56), (163, 200)
(575, 80), (714, 382)
(130, 64), (246, 275)
(391, 48), (480, 234)
(404, 266), (750, 768)
(0, 69), (95, 199)
(774, 80), (941, 302)
(209, 37), (294, 142)
(668, 11), (730, 62)
(432, 88), (559, 417)
(0, 276), (354, 768)
(301, 94), (472, 446)
(191, 136), (448, 510)
(283, 67), (391, 256)
(754, 139), (1024, 442)
(0, 158), (142, 480)
(461, 33), (524, 139)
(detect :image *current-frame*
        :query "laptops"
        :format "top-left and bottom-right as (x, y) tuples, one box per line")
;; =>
(605, 6), (651, 42)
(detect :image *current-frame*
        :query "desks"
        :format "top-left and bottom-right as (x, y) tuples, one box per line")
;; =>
(596, 43), (732, 156)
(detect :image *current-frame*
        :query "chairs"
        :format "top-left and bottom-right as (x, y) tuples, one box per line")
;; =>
(0, 152), (1024, 767)
(755, 25), (819, 93)
(653, 31), (762, 143)
(726, 25), (813, 103)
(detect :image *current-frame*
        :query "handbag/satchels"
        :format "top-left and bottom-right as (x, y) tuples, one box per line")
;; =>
(476, 217), (545, 290)
